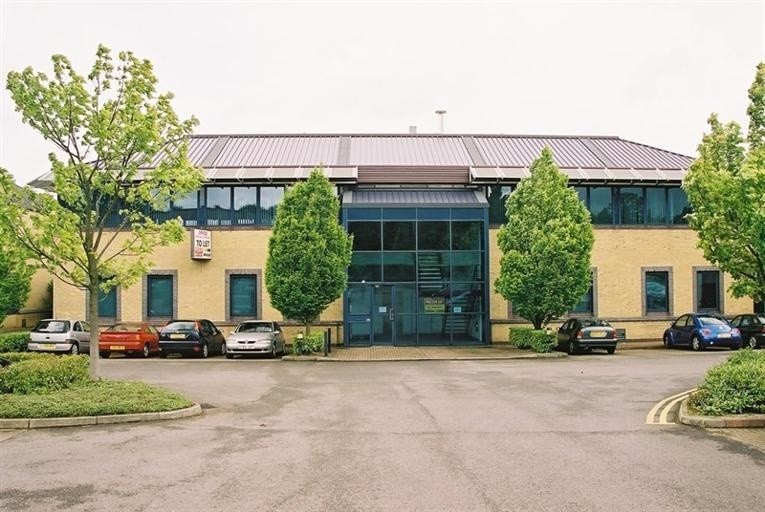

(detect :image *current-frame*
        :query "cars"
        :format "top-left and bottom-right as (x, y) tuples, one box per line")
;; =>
(728, 314), (765, 350)
(160, 319), (226, 359)
(646, 282), (666, 299)
(99, 322), (161, 358)
(225, 320), (287, 358)
(27, 318), (102, 356)
(557, 318), (617, 355)
(662, 312), (742, 351)
(447, 290), (481, 311)
(432, 280), (483, 299)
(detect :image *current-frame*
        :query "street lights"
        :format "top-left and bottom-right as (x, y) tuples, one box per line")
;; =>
(434, 110), (448, 133)
